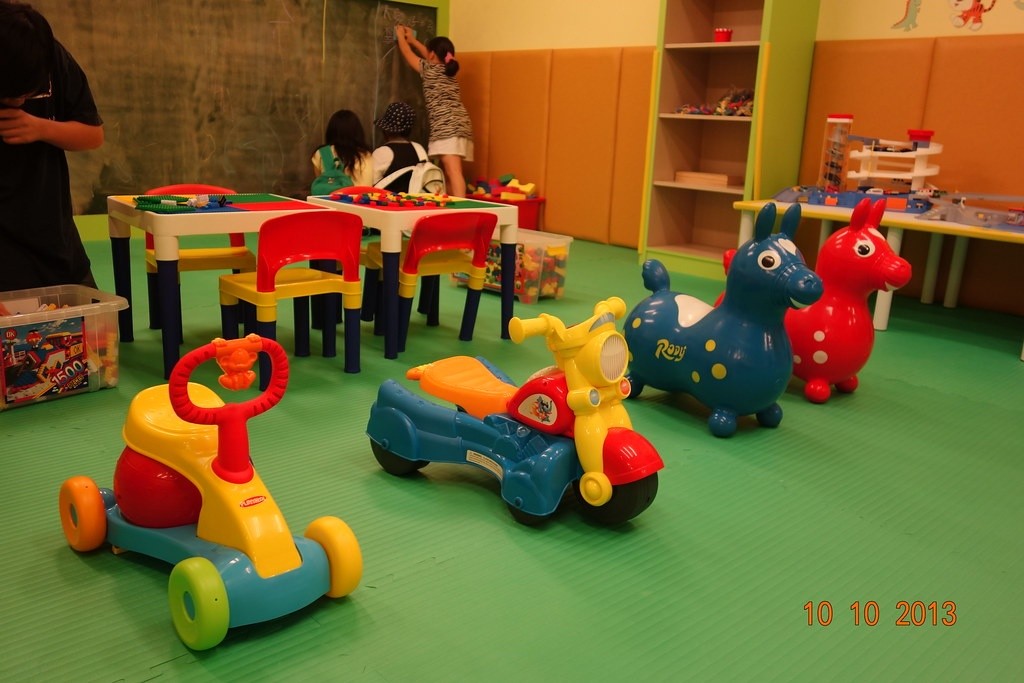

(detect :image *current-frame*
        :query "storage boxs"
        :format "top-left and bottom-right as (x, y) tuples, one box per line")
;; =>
(0, 284), (128, 410)
(485, 227), (572, 306)
(468, 183), (546, 232)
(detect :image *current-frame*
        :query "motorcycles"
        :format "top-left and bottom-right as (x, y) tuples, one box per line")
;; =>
(58, 333), (364, 650)
(364, 298), (665, 526)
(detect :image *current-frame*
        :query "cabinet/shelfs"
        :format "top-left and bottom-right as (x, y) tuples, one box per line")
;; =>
(636, 0), (819, 283)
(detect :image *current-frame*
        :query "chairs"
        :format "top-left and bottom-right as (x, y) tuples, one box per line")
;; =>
(219, 209), (364, 381)
(145, 181), (257, 331)
(362, 213), (501, 353)
(330, 185), (393, 322)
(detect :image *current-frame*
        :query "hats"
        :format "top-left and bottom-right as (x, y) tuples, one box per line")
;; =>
(372, 102), (416, 132)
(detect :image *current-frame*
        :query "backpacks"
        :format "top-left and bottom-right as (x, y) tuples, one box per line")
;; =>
(375, 142), (447, 196)
(311, 146), (354, 196)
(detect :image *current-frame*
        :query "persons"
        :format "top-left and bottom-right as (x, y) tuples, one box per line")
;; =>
(396, 25), (474, 199)
(312, 101), (429, 238)
(0, 0), (104, 323)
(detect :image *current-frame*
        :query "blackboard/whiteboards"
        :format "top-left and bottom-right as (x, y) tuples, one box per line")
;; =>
(3, 0), (437, 216)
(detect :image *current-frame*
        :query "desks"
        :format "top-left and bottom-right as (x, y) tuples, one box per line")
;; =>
(307, 194), (517, 357)
(106, 194), (327, 379)
(730, 195), (1024, 361)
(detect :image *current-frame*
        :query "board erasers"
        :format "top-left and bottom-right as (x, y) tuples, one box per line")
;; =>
(393, 26), (418, 42)
